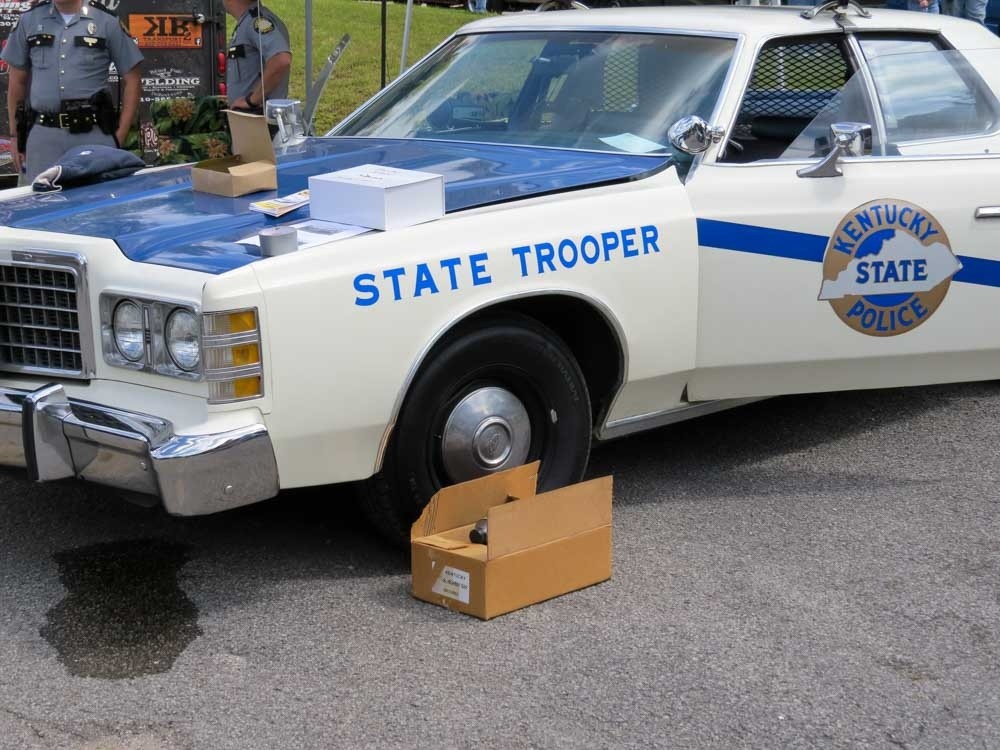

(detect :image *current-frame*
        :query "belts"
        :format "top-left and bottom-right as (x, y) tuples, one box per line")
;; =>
(30, 109), (98, 129)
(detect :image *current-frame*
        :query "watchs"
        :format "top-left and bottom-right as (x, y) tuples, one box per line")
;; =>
(245, 91), (260, 107)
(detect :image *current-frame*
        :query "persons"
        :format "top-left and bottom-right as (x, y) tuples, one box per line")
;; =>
(466, 0), (487, 14)
(786, 0), (990, 26)
(0, 0), (145, 187)
(223, 0), (292, 140)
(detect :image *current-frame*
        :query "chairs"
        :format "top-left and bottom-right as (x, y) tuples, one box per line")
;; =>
(575, 112), (670, 149)
(722, 116), (814, 164)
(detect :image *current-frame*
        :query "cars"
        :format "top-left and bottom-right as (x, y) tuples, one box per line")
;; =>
(0, 0), (1000, 556)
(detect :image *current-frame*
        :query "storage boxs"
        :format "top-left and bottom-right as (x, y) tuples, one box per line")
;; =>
(410, 460), (613, 620)
(308, 164), (445, 231)
(190, 109), (277, 198)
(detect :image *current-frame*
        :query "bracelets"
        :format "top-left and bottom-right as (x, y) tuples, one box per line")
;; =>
(10, 133), (18, 138)
